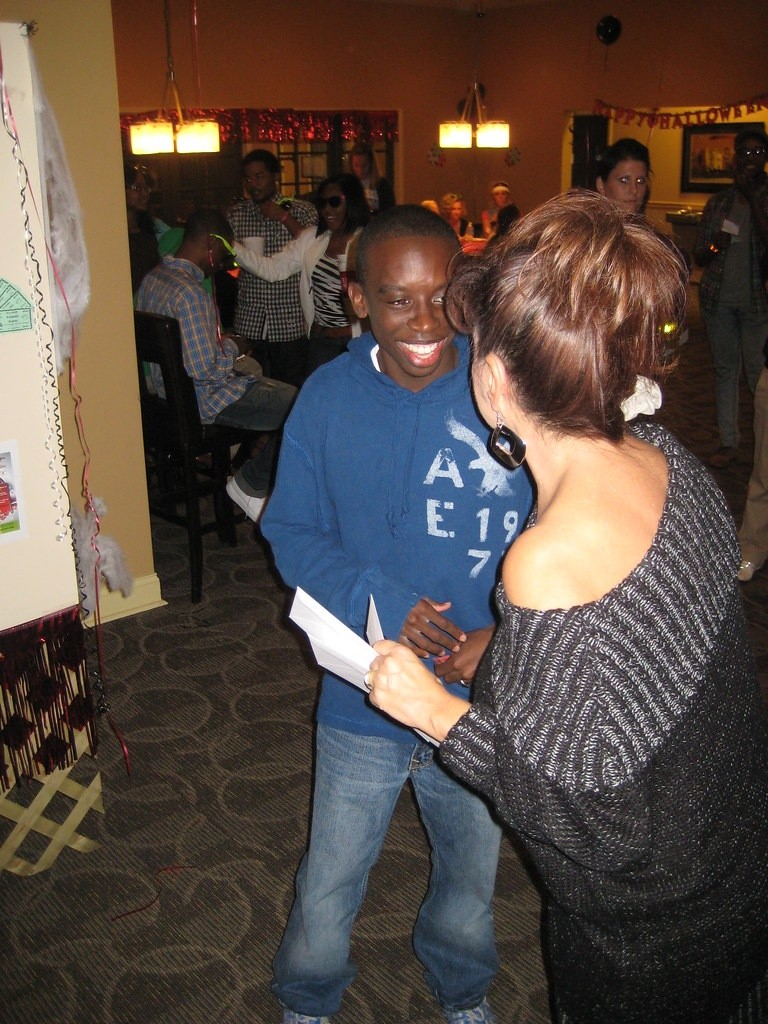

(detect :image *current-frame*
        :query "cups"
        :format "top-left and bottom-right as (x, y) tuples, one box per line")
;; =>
(243, 237), (264, 257)
(472, 223), (483, 237)
(458, 218), (468, 236)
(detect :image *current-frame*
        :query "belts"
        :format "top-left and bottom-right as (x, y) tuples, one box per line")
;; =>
(312, 324), (352, 338)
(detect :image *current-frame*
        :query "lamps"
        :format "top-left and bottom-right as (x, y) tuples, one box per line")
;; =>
(438, 8), (511, 148)
(130, 1), (221, 154)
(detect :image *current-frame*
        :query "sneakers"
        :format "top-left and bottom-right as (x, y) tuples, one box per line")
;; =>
(446, 1002), (496, 1024)
(282, 1006), (330, 1024)
(736, 560), (762, 582)
(226, 476), (271, 525)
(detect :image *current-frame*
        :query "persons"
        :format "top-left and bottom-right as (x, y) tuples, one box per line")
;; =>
(123, 150), (518, 526)
(588, 137), (653, 220)
(736, 336), (768, 582)
(364, 187), (768, 1024)
(693, 125), (768, 466)
(259, 204), (537, 1024)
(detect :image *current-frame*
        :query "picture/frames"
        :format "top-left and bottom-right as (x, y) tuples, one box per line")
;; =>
(680, 121), (765, 193)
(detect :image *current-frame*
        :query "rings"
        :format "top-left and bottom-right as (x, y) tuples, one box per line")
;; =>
(460, 680), (471, 688)
(364, 671), (373, 688)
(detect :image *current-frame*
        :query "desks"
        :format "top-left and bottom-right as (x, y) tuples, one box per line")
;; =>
(667, 210), (701, 269)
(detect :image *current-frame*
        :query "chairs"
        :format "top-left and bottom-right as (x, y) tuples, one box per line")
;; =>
(133, 309), (263, 605)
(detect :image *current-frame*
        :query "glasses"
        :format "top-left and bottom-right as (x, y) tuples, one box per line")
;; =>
(315, 195), (346, 209)
(210, 233), (237, 268)
(127, 186), (152, 193)
(738, 146), (767, 158)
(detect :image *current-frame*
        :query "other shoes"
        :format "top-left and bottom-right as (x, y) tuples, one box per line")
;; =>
(229, 442), (241, 460)
(709, 446), (737, 468)
